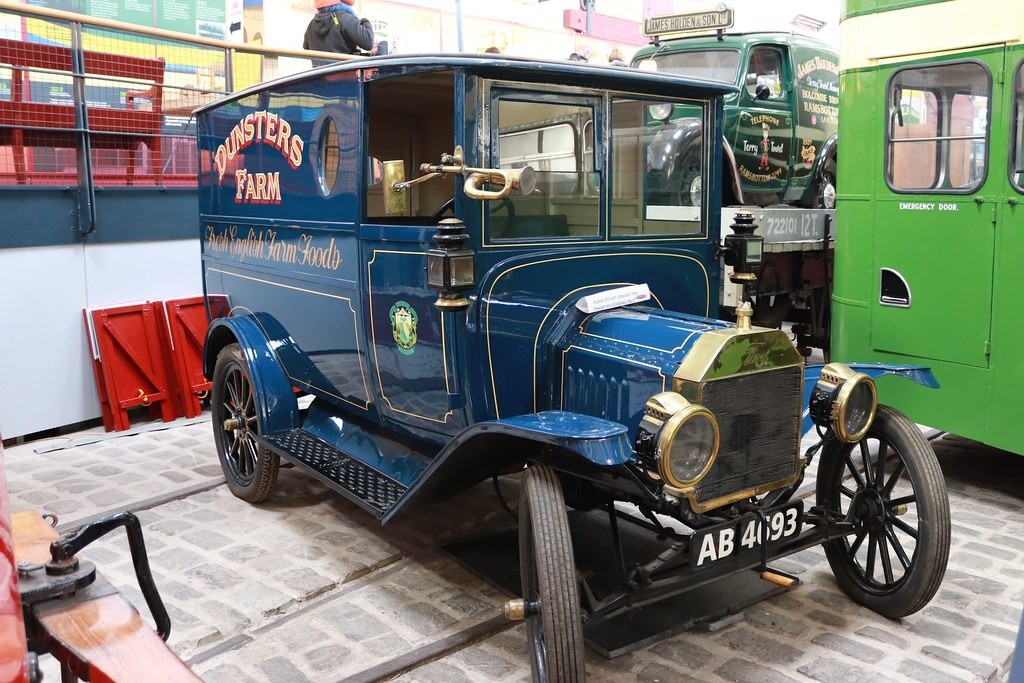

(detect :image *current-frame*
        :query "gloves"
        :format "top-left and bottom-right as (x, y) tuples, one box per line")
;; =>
(361, 19), (370, 25)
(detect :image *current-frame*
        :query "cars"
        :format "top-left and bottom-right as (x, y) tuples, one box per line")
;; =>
(192, 53), (951, 682)
(632, 1), (921, 210)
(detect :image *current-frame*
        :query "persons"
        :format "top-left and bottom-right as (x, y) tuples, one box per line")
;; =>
(485, 47), (501, 55)
(303, 0), (389, 68)
(568, 46), (592, 63)
(606, 48), (629, 67)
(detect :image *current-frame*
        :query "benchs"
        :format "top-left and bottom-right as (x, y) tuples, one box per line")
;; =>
(0, 37), (166, 185)
(367, 215), (569, 241)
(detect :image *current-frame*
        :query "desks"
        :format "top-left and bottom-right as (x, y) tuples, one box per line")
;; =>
(160, 104), (209, 175)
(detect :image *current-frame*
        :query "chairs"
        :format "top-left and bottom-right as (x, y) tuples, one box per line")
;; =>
(757, 74), (783, 99)
